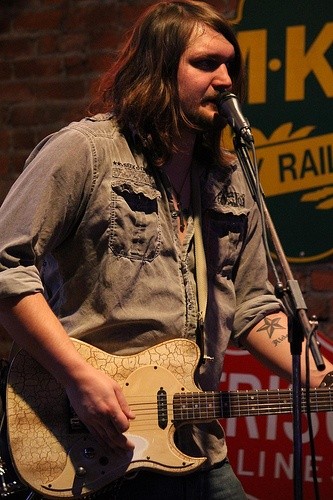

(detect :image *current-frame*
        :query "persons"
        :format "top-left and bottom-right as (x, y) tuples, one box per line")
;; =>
(0, 0), (333, 500)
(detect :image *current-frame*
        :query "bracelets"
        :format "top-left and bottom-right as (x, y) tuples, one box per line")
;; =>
(318, 371), (333, 389)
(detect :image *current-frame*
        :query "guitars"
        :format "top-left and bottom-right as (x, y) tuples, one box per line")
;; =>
(5, 336), (333, 498)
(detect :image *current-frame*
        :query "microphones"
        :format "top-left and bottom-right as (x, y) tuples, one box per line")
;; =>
(218, 91), (254, 145)
